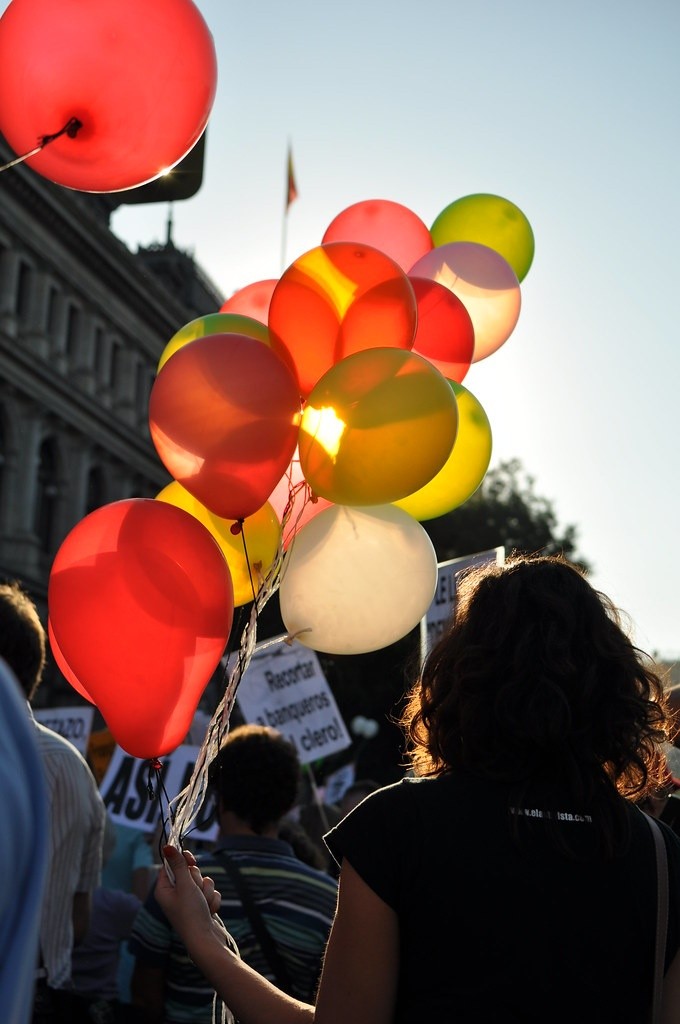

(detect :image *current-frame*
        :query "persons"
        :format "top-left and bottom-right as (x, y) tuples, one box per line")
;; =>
(153, 555), (680, 1024)
(0, 588), (393, 1024)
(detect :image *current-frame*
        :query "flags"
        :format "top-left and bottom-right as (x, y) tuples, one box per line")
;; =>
(286, 156), (297, 207)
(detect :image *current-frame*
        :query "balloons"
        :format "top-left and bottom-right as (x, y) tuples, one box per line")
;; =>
(48, 499), (234, 758)
(0, 0), (217, 193)
(148, 192), (534, 652)
